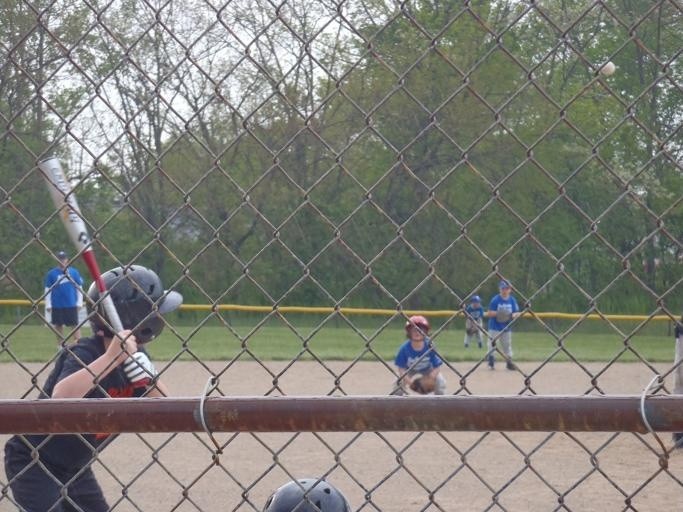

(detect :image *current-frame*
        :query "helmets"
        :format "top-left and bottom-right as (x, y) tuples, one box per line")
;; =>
(264, 477), (351, 512)
(85, 263), (184, 344)
(404, 315), (430, 333)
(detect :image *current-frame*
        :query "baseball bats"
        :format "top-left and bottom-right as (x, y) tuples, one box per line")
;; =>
(40, 156), (124, 335)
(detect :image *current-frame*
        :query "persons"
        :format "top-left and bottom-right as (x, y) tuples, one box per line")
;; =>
(463, 295), (483, 348)
(4, 264), (182, 512)
(486, 280), (519, 369)
(670, 317), (683, 449)
(44, 250), (83, 350)
(390, 315), (446, 395)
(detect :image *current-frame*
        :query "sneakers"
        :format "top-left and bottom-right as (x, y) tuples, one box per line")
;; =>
(506, 361), (515, 370)
(488, 362), (495, 369)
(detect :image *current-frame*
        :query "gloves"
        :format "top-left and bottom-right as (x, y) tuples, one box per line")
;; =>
(122, 351), (161, 389)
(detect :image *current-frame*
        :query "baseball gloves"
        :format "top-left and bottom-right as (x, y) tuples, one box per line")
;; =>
(494, 310), (512, 325)
(409, 373), (438, 391)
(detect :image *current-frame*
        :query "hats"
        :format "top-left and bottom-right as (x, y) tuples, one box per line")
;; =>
(58, 251), (66, 258)
(470, 296), (481, 303)
(499, 280), (512, 289)
